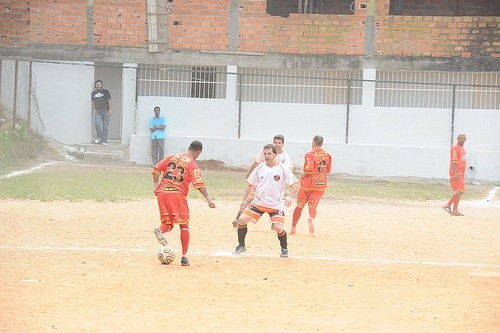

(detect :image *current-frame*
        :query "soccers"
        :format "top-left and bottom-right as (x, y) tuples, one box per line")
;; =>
(157, 246), (176, 262)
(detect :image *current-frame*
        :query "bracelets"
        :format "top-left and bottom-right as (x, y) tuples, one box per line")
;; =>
(154, 183), (159, 190)
(205, 195), (211, 204)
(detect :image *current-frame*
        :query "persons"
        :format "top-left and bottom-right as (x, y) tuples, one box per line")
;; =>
(150, 140), (216, 266)
(232, 144), (300, 258)
(91, 80), (112, 146)
(148, 106), (167, 166)
(442, 133), (468, 216)
(232, 134), (293, 231)
(290, 135), (332, 235)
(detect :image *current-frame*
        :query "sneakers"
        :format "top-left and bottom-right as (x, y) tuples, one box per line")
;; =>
(232, 245), (247, 254)
(280, 248), (288, 257)
(154, 228), (168, 246)
(181, 257), (189, 265)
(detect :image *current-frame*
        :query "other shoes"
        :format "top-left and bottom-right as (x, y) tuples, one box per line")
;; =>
(94, 137), (101, 143)
(102, 143), (107, 145)
(289, 227), (296, 235)
(308, 218), (314, 234)
